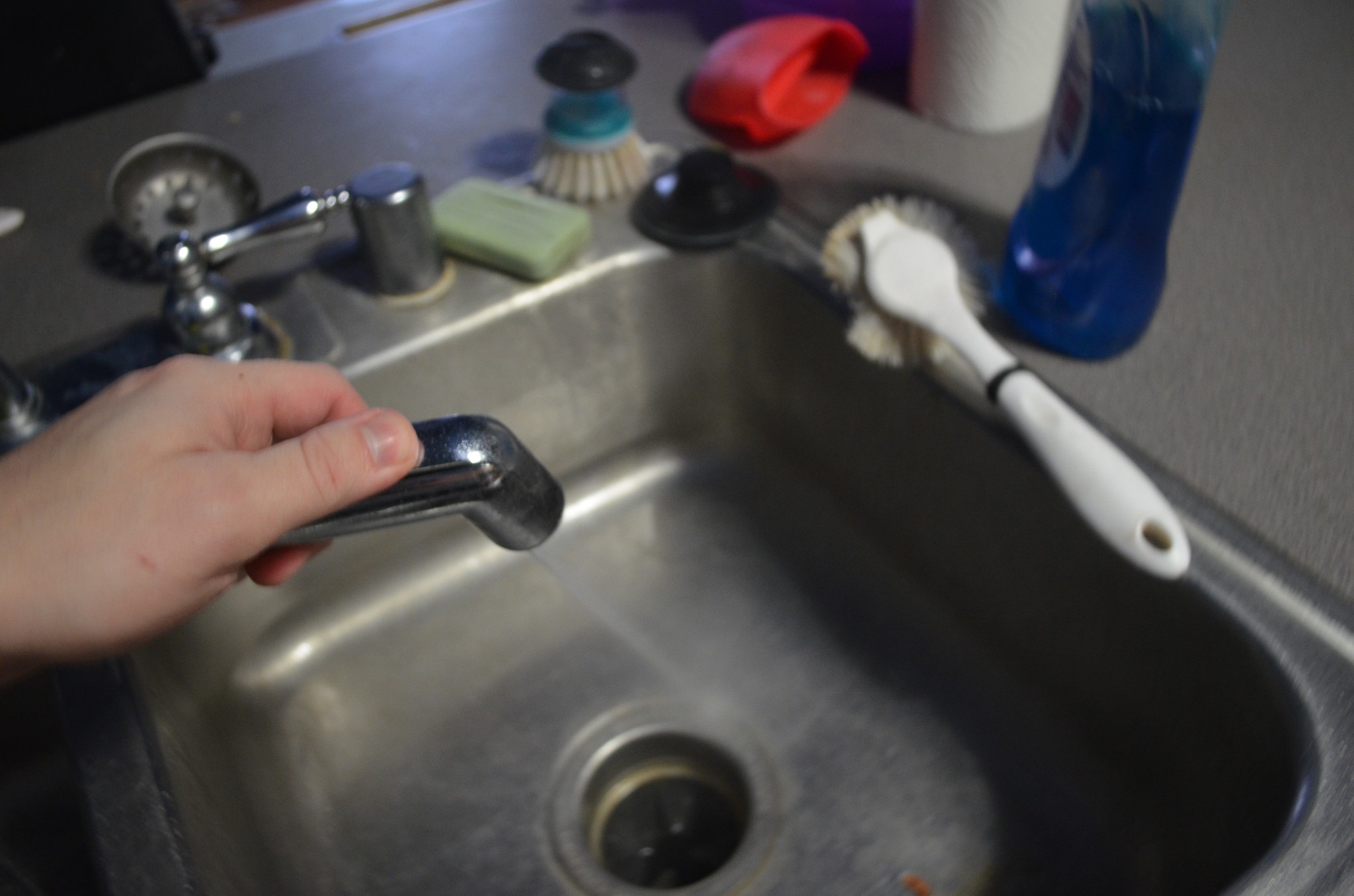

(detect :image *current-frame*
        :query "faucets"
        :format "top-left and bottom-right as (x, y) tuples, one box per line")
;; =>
(260, 412), (568, 551)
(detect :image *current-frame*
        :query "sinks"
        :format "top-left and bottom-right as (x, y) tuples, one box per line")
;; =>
(0, 141), (1354, 896)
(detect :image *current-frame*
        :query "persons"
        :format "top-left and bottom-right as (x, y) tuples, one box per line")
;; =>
(0, 350), (423, 686)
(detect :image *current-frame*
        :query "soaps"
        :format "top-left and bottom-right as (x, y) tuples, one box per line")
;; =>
(421, 174), (603, 281)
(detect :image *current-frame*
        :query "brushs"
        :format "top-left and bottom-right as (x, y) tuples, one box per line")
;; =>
(519, 30), (649, 204)
(817, 192), (1197, 583)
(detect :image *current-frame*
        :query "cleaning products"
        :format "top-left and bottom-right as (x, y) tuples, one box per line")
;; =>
(985, 1), (1222, 364)
